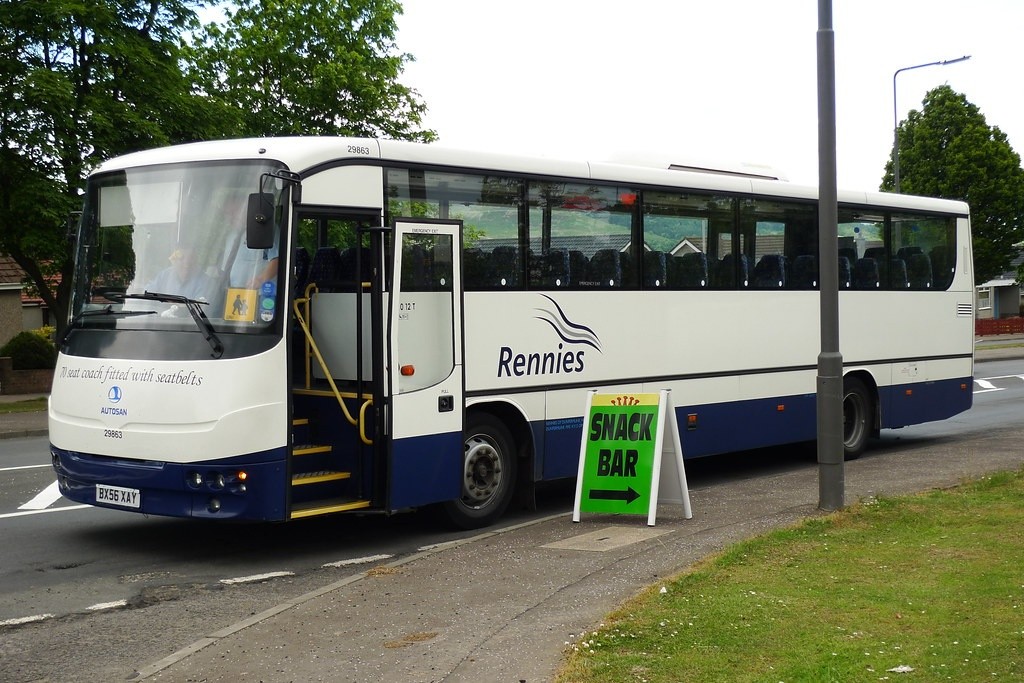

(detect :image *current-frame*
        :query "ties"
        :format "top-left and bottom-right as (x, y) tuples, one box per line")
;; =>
(224, 230), (245, 288)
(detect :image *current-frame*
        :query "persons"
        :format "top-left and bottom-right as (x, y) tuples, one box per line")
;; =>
(143, 243), (217, 315)
(220, 194), (281, 298)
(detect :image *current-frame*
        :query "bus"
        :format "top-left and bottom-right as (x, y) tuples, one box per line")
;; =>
(46, 136), (977, 526)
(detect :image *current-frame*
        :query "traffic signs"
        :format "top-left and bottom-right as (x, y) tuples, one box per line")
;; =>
(572, 389), (693, 526)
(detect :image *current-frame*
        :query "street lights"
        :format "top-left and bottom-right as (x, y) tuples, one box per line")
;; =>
(893, 56), (973, 254)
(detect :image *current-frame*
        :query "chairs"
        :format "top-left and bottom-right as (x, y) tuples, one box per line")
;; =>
(292, 247), (951, 337)
